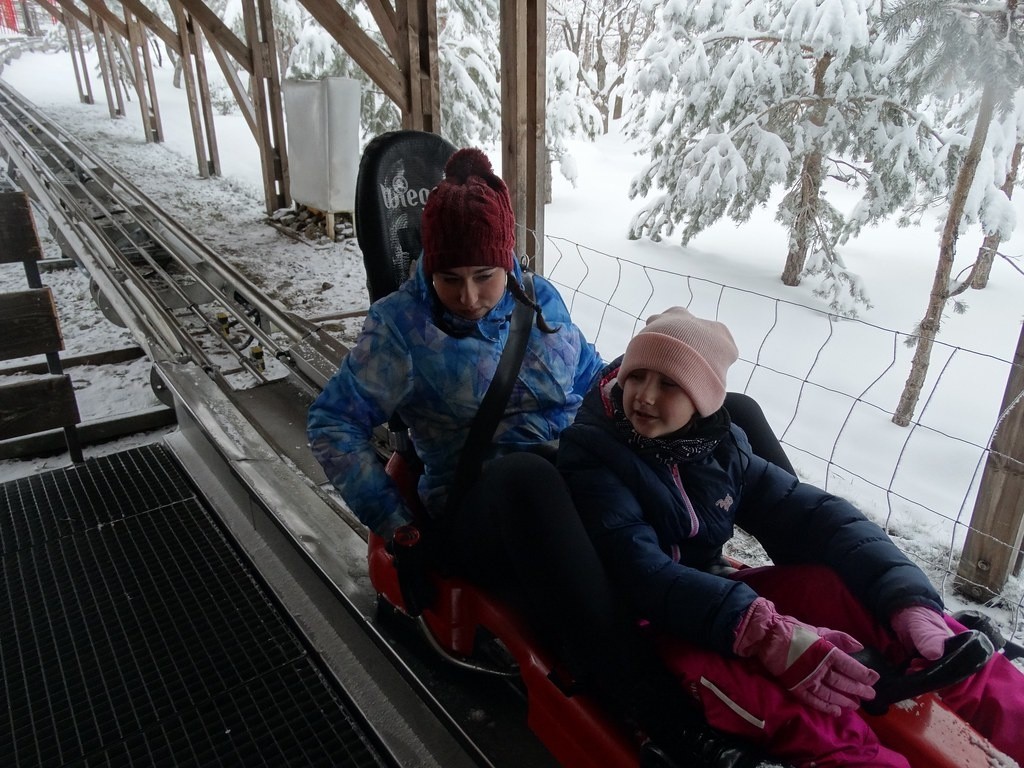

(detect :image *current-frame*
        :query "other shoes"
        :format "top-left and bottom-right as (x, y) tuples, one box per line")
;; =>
(653, 707), (744, 768)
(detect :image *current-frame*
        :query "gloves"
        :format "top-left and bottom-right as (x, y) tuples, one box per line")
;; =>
(381, 520), (463, 616)
(890, 605), (979, 704)
(734, 596), (881, 718)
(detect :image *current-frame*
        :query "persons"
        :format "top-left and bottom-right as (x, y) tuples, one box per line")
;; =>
(305, 148), (1024, 768)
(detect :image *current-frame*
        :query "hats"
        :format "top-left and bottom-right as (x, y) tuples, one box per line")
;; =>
(420, 146), (515, 273)
(617, 306), (737, 418)
